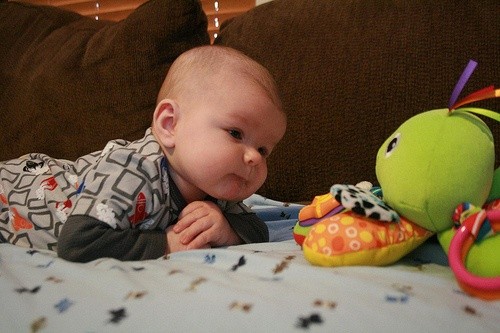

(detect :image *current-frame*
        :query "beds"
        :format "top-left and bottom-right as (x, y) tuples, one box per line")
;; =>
(0, 193), (500, 333)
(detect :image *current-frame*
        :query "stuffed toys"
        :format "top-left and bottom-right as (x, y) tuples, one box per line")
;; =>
(299, 58), (500, 302)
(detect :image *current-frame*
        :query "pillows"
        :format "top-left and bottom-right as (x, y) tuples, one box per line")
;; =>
(216, 0), (500, 203)
(0, 1), (211, 161)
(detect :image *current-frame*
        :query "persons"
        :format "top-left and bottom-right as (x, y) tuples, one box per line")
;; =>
(1, 43), (287, 267)
